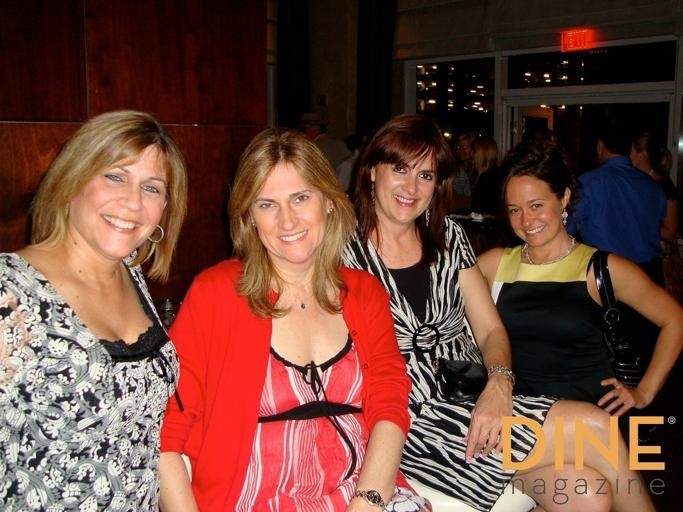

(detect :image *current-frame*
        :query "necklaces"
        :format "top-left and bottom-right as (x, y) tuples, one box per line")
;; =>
(275, 278), (319, 308)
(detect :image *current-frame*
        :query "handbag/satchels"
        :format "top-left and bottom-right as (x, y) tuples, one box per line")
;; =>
(433, 357), (488, 414)
(604, 308), (640, 388)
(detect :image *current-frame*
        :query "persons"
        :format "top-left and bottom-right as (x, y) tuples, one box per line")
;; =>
(0, 110), (188, 512)
(159, 124), (411, 512)
(456, 129), (680, 289)
(341, 112), (658, 512)
(401, 145), (683, 512)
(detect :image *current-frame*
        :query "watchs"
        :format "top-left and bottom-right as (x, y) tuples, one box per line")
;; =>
(353, 489), (386, 509)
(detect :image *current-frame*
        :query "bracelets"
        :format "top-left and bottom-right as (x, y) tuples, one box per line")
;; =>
(488, 365), (515, 385)
